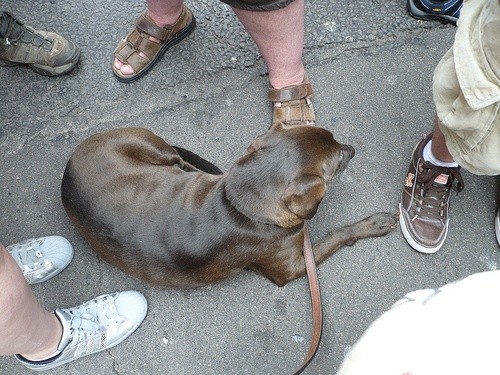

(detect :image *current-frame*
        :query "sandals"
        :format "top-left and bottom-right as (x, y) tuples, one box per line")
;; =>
(267, 72), (316, 128)
(407, 0), (464, 26)
(112, 3), (196, 84)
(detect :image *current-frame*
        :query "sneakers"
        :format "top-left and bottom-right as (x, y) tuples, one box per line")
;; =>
(0, 11), (81, 77)
(494, 207), (500, 246)
(399, 132), (464, 254)
(13, 291), (147, 371)
(6, 235), (73, 284)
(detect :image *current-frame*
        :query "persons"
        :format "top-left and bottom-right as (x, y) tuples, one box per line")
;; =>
(0, 10), (81, 75)
(112, 0), (317, 127)
(407, 0), (463, 25)
(398, 0), (500, 253)
(0, 234), (148, 371)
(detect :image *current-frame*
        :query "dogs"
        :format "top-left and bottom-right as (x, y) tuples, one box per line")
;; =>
(61, 120), (397, 289)
